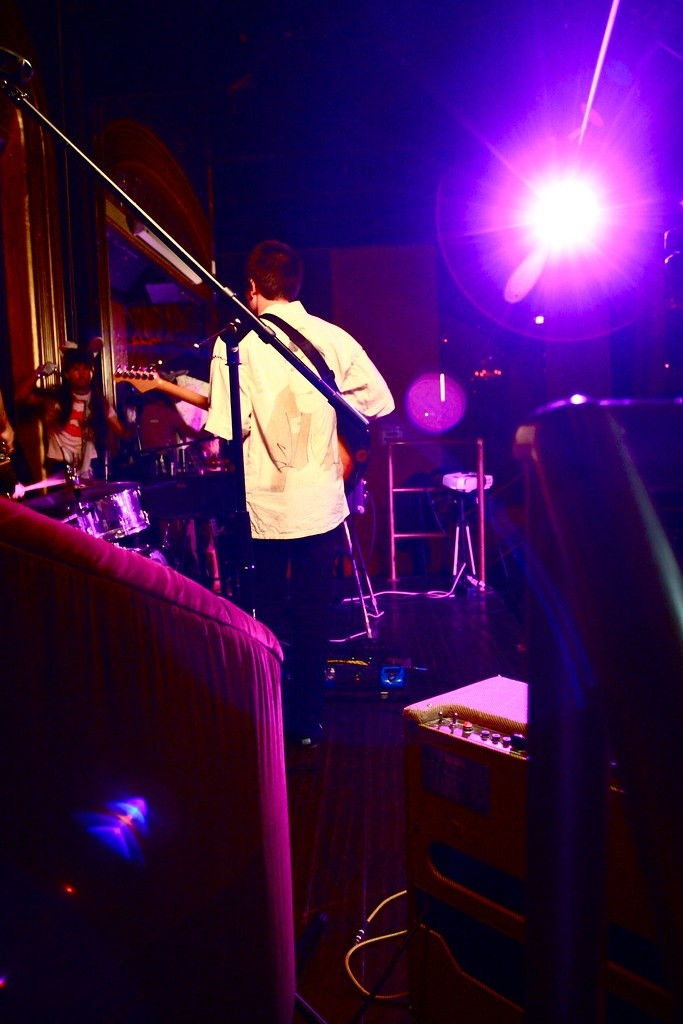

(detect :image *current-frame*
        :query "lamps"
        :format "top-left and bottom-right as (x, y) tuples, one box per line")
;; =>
(131, 219), (203, 286)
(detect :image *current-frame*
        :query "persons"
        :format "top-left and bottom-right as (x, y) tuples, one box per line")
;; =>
(0, 347), (209, 498)
(205, 239), (394, 688)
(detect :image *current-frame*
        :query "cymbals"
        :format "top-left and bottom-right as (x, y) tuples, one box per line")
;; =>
(19, 480), (139, 508)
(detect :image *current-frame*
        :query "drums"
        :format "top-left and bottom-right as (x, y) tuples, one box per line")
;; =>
(48, 487), (150, 540)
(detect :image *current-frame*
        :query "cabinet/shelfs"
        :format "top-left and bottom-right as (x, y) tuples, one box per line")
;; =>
(388, 436), (484, 587)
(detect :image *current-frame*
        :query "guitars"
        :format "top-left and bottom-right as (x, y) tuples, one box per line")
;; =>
(113, 365), (368, 498)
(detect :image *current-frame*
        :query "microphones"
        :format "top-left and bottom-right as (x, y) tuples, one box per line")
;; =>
(0, 46), (33, 81)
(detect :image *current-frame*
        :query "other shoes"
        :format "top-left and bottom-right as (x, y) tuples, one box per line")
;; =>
(296, 720), (328, 751)
